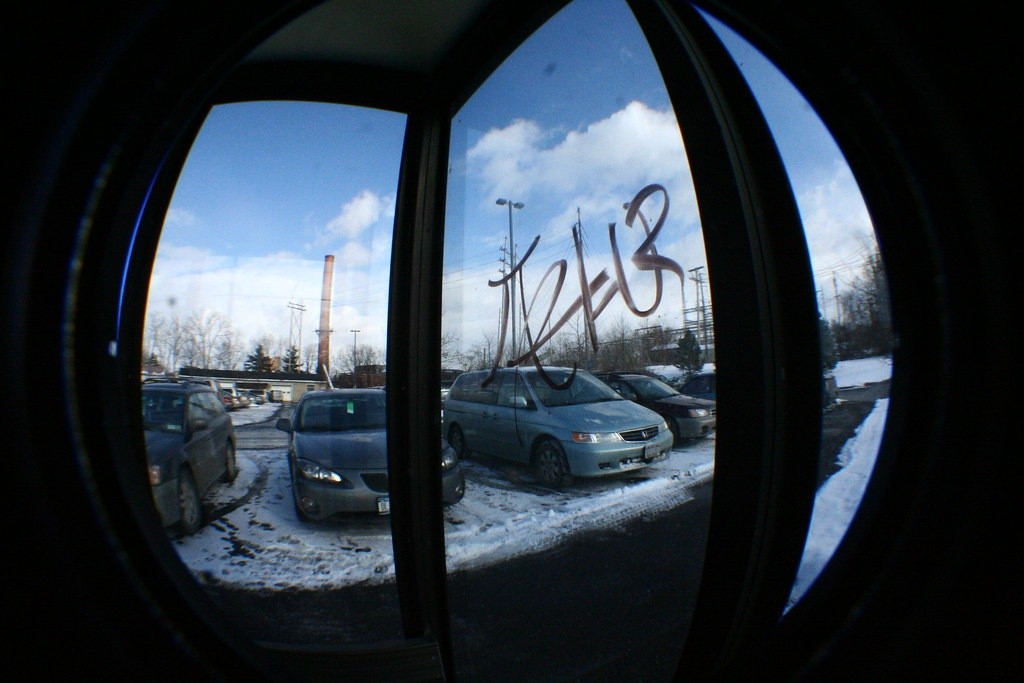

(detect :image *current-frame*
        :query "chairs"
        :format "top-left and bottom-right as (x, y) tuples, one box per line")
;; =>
(303, 406), (331, 428)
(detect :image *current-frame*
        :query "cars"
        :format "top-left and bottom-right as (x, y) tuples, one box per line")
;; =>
(591, 368), (716, 447)
(670, 370), (716, 402)
(207, 373), (292, 412)
(275, 387), (465, 520)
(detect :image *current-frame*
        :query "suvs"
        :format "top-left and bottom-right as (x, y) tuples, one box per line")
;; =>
(445, 364), (674, 491)
(140, 376), (237, 535)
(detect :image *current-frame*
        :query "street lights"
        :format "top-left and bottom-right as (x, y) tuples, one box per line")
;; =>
(497, 198), (526, 360)
